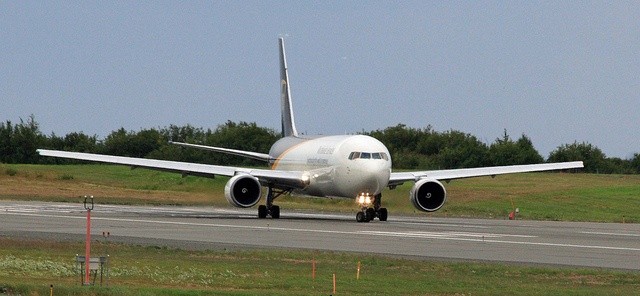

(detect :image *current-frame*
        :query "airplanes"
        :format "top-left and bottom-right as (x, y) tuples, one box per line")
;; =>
(36, 36), (586, 222)
